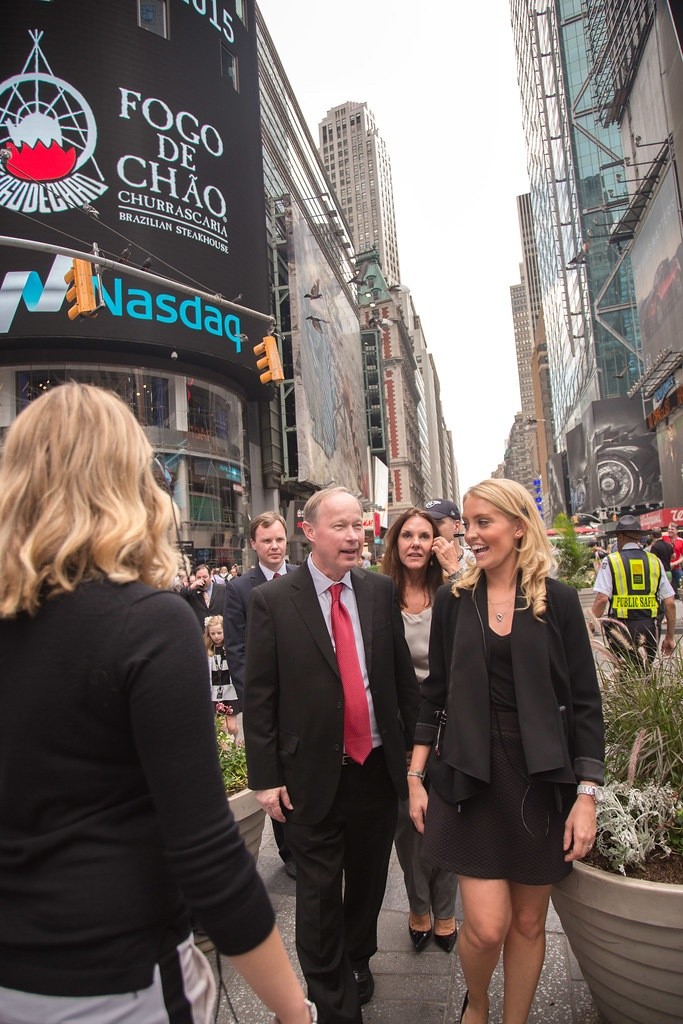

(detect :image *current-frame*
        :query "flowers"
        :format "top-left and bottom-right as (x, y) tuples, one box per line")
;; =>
(211, 700), (249, 794)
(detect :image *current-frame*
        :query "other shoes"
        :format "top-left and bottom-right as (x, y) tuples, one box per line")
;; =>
(458, 989), (469, 1024)
(284, 861), (297, 880)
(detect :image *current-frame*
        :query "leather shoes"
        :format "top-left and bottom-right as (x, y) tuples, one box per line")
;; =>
(352, 959), (374, 1005)
(434, 925), (457, 953)
(409, 909), (432, 950)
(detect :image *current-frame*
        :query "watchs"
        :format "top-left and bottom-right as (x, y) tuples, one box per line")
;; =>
(301, 997), (318, 1023)
(577, 784), (605, 804)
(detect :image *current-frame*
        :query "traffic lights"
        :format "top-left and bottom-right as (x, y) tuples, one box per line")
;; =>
(253, 335), (285, 384)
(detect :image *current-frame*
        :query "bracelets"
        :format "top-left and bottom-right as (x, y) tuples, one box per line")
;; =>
(408, 771), (425, 780)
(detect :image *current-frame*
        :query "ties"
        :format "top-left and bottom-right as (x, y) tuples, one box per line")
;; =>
(272, 573), (281, 578)
(328, 583), (373, 765)
(203, 592), (209, 608)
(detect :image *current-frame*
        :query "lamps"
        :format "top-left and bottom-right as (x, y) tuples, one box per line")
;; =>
(293, 192), (357, 265)
(586, 135), (671, 242)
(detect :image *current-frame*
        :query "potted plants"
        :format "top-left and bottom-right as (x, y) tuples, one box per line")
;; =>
(548, 513), (610, 636)
(549, 611), (683, 1024)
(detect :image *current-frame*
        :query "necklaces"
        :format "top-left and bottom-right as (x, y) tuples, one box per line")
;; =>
(485, 584), (515, 621)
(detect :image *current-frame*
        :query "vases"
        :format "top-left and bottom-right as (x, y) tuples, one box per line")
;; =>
(193, 787), (265, 954)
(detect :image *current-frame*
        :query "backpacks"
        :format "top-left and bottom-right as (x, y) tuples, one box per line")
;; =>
(218, 573), (229, 583)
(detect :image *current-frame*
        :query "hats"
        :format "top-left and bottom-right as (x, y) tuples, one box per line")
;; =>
(605, 515), (652, 535)
(421, 498), (461, 522)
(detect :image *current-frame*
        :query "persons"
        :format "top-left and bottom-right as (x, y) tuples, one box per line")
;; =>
(201, 615), (243, 744)
(168, 514), (682, 629)
(0, 376), (318, 1024)
(378, 497), (480, 956)
(591, 515), (677, 683)
(222, 511), (302, 715)
(406, 476), (609, 1024)
(241, 487), (422, 1024)
